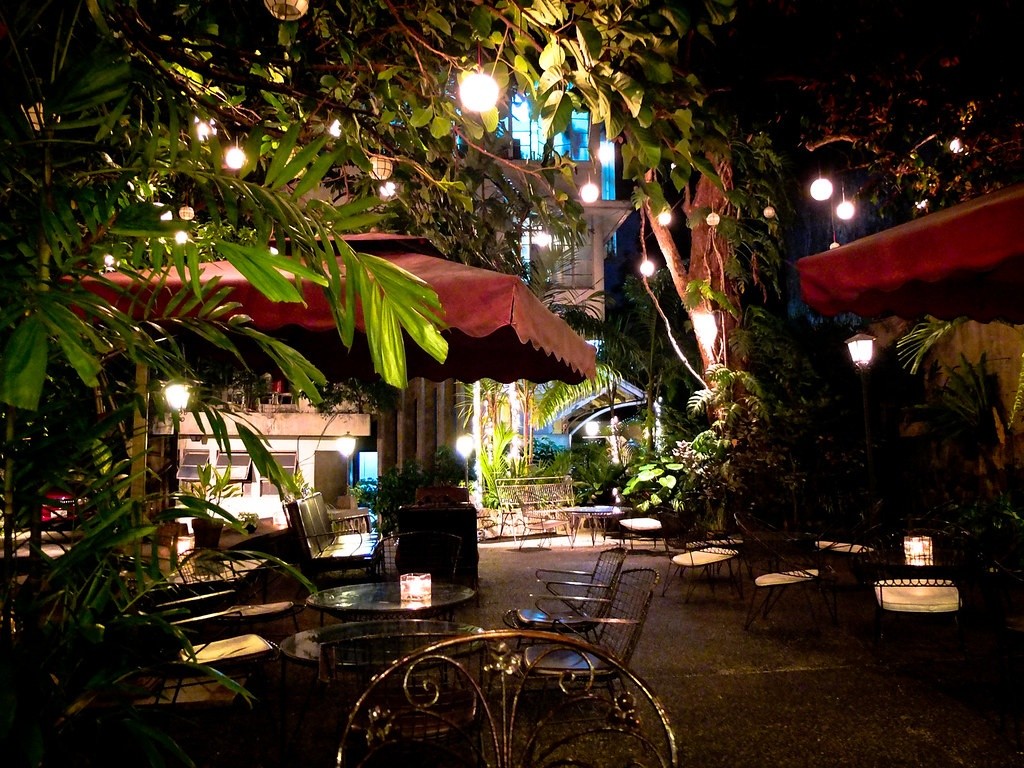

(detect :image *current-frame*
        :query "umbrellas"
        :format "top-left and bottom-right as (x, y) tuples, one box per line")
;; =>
(792, 181), (1024, 330)
(44, 222), (599, 579)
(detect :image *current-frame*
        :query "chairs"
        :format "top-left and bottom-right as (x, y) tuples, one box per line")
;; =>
(124, 489), (971, 768)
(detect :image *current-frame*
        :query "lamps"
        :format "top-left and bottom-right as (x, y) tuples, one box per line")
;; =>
(163, 378), (193, 415)
(335, 431), (360, 496)
(457, 434), (476, 504)
(96, 69), (968, 273)
